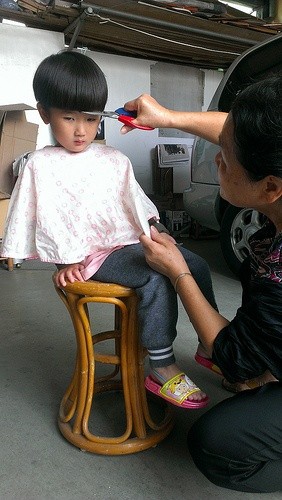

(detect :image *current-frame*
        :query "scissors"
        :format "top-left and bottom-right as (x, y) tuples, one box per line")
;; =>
(82, 105), (156, 130)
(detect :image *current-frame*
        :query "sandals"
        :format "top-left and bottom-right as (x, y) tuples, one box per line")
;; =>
(195, 351), (223, 375)
(145, 369), (210, 410)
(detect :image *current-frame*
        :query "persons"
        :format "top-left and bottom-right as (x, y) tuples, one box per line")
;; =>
(120, 73), (282, 493)
(1, 50), (223, 409)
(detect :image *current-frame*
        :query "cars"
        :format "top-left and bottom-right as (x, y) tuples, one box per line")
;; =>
(182, 29), (282, 280)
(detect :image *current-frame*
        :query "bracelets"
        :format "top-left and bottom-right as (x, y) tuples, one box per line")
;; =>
(174, 273), (191, 292)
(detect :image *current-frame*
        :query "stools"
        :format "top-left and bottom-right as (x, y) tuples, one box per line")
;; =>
(55, 278), (176, 456)
